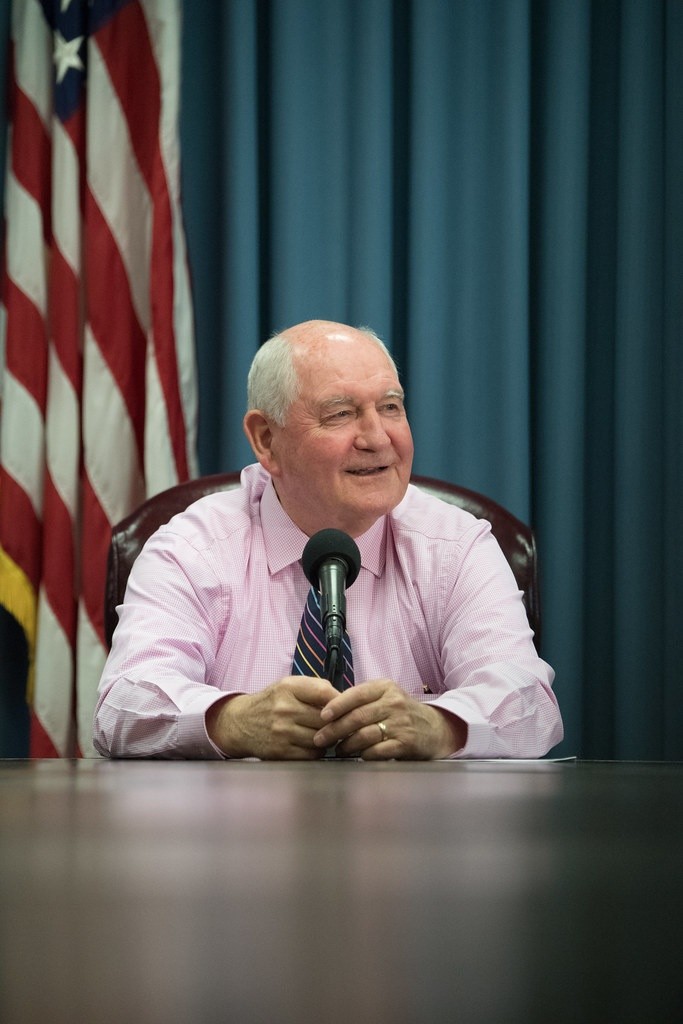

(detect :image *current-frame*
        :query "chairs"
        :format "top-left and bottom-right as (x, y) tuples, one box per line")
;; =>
(100, 468), (540, 657)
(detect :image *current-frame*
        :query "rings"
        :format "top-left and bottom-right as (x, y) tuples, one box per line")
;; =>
(375, 722), (388, 741)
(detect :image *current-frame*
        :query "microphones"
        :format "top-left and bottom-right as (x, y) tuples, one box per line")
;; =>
(301, 527), (362, 653)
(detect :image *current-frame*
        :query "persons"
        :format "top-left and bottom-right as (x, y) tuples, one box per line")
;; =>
(93, 322), (564, 761)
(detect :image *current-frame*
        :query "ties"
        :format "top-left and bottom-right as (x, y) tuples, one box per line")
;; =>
(291, 584), (355, 694)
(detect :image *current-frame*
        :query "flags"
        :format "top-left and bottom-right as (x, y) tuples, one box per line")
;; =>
(0, 0), (205, 760)
